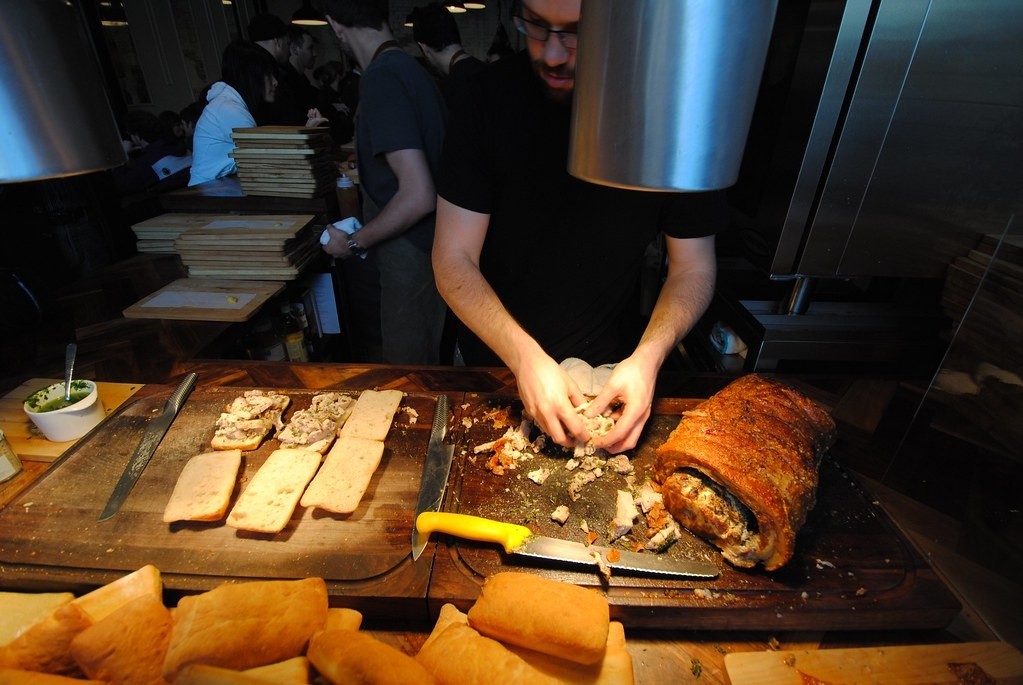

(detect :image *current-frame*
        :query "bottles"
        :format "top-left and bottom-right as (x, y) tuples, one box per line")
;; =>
(252, 302), (315, 362)
(336, 173), (362, 223)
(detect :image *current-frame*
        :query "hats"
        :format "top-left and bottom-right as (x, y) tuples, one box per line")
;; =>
(247, 14), (287, 41)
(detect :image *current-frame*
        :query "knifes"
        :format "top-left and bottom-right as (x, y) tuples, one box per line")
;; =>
(415, 511), (719, 577)
(412, 393), (454, 561)
(98, 373), (199, 522)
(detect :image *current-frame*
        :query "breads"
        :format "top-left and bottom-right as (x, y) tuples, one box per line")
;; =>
(654, 373), (835, 572)
(0, 565), (634, 685)
(163, 389), (404, 534)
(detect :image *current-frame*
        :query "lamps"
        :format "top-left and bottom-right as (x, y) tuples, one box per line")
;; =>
(0, 0), (487, 185)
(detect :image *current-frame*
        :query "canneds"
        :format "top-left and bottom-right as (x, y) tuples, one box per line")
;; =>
(0, 429), (23, 485)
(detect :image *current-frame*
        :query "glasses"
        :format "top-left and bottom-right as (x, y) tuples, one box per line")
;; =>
(512, 2), (579, 48)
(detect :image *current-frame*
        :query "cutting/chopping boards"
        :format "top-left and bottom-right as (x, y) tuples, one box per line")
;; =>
(0, 380), (910, 592)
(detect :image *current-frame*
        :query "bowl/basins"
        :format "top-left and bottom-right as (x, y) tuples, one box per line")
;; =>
(24, 379), (106, 442)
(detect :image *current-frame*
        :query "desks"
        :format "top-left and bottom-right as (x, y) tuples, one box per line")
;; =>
(121, 223), (325, 357)
(0, 359), (1023, 685)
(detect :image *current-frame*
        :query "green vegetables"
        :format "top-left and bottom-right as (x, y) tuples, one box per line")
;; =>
(22, 380), (91, 413)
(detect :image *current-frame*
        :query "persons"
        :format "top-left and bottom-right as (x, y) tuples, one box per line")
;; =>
(158, 110), (186, 157)
(316, 61), (346, 102)
(317, 0), (448, 367)
(269, 24), (318, 126)
(180, 102), (206, 137)
(115, 112), (149, 159)
(431, 0), (727, 454)
(413, 4), (483, 102)
(187, 40), (329, 188)
(246, 13), (292, 69)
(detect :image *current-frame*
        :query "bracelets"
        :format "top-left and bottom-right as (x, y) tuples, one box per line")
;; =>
(347, 234), (366, 256)
